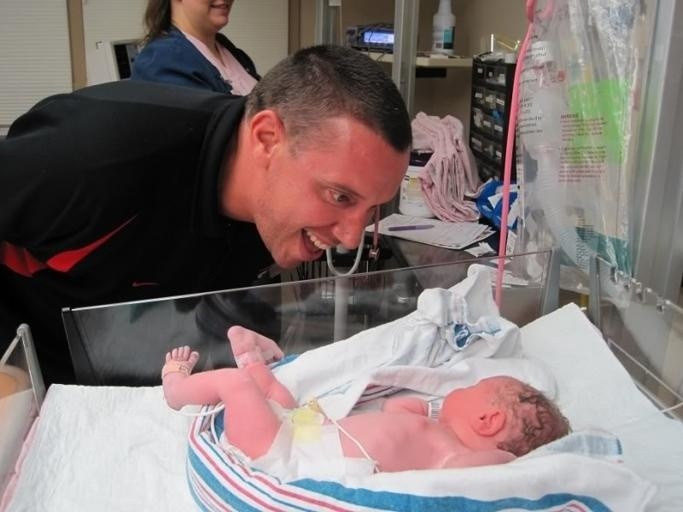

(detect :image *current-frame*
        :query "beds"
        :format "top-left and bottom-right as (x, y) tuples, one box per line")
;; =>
(0, 250), (682, 512)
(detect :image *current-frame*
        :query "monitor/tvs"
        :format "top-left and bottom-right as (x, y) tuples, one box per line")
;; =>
(110, 37), (148, 81)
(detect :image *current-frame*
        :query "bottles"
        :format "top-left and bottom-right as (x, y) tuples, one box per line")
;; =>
(399, 149), (439, 218)
(431, 0), (456, 56)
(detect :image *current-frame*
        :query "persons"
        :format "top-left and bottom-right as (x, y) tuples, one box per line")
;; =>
(0, 41), (415, 404)
(130, 1), (263, 102)
(159, 323), (574, 476)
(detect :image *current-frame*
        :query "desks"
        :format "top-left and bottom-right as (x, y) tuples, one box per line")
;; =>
(384, 236), (583, 328)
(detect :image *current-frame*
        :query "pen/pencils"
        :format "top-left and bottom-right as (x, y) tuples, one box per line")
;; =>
(388, 225), (434, 231)
